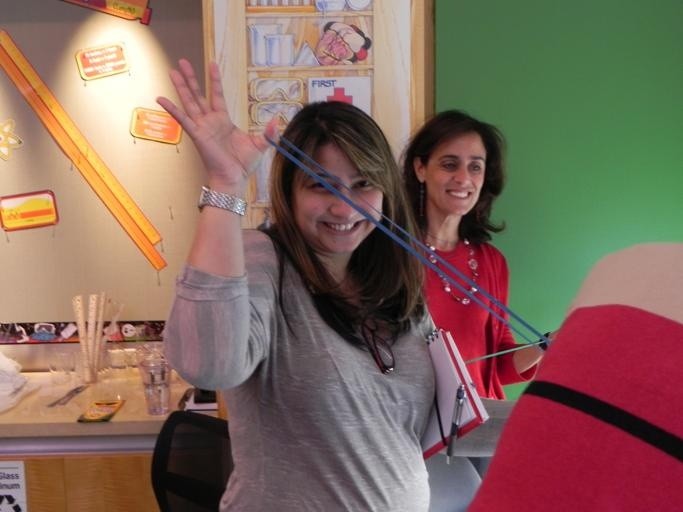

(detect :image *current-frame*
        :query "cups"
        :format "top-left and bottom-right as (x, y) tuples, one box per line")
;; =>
(135, 345), (171, 415)
(124, 342), (136, 367)
(106, 343), (127, 370)
(245, 24), (281, 65)
(264, 33), (294, 67)
(47, 351), (74, 383)
(77, 351), (102, 384)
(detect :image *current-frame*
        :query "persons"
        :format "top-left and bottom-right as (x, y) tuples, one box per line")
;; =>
(158, 58), (434, 512)
(399, 110), (562, 400)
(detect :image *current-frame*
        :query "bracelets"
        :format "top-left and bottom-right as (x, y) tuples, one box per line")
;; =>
(539, 332), (548, 350)
(197, 186), (247, 216)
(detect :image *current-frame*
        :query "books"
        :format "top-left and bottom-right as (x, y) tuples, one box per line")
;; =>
(421, 326), (490, 461)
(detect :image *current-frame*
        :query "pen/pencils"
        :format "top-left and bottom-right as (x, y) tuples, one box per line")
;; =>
(446, 384), (467, 465)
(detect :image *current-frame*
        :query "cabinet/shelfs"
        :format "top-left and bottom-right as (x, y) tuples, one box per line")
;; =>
(0, 371), (215, 512)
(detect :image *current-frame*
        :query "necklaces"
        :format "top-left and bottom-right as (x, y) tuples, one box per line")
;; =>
(421, 237), (479, 305)
